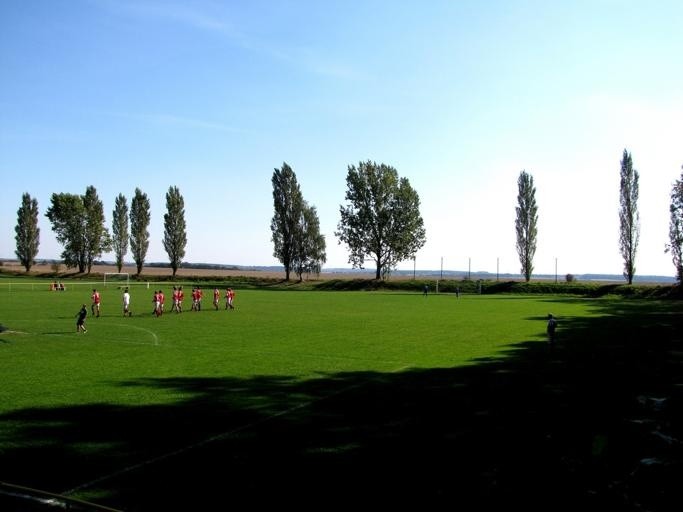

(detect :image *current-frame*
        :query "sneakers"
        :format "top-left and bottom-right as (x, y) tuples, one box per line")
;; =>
(96, 315), (99, 318)
(83, 330), (87, 333)
(129, 312), (132, 317)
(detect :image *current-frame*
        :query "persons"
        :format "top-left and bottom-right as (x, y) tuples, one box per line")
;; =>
(90, 289), (101, 317)
(546, 313), (558, 354)
(72, 303), (88, 334)
(151, 287), (236, 318)
(422, 284), (428, 297)
(122, 289), (132, 316)
(47, 280), (64, 291)
